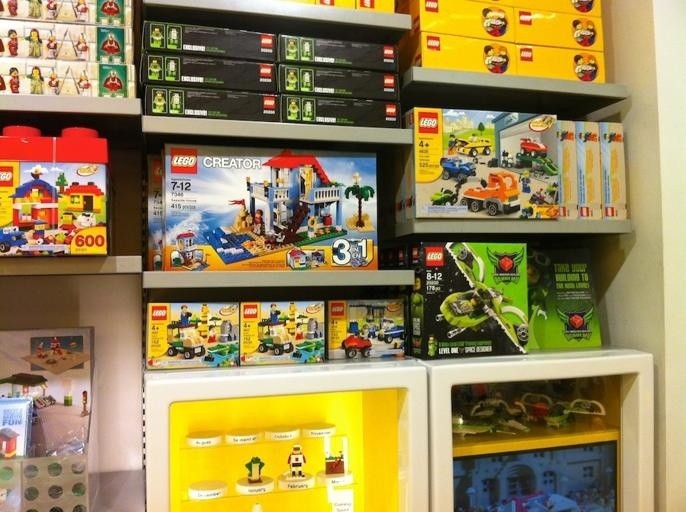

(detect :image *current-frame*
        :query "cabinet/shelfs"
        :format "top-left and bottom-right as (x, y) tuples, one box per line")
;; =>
(0, 0), (656, 511)
